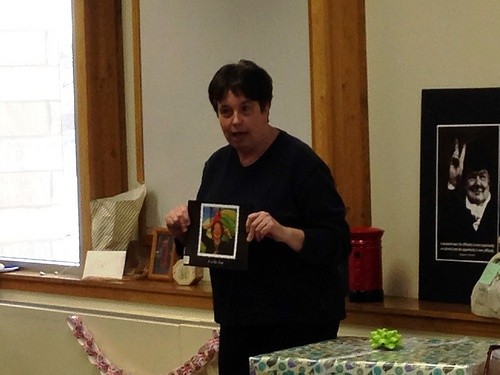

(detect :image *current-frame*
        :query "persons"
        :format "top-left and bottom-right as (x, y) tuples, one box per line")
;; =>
(202, 217), (234, 254)
(166, 59), (352, 375)
(438, 137), (498, 245)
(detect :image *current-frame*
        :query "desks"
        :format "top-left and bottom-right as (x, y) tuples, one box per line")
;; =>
(249, 336), (500, 375)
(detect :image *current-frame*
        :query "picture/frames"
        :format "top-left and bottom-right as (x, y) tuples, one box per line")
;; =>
(148, 228), (176, 281)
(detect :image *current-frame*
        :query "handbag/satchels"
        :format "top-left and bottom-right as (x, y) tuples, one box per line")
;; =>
(89, 184), (148, 250)
